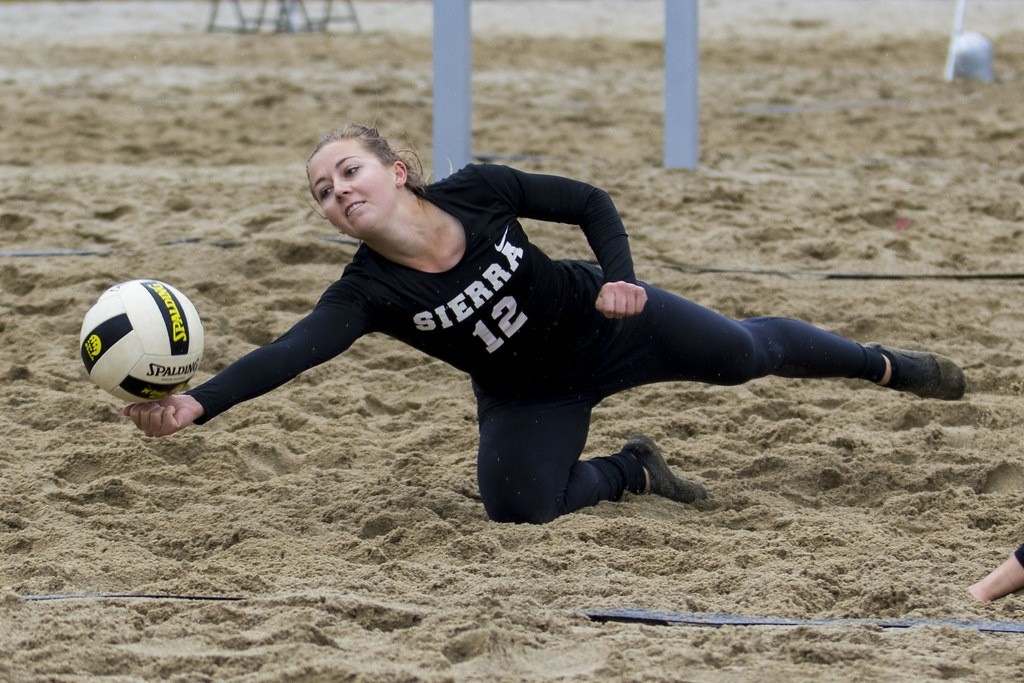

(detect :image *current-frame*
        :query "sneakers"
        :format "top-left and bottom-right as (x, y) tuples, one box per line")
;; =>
(866, 338), (966, 400)
(622, 433), (708, 507)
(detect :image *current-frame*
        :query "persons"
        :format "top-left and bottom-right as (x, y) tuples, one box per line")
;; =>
(123, 124), (968, 526)
(966, 532), (1024, 609)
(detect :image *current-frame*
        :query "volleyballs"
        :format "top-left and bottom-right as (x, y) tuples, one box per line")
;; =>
(79, 277), (205, 404)
